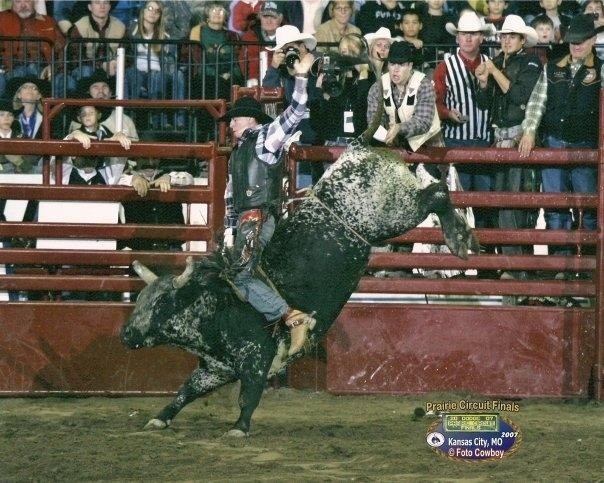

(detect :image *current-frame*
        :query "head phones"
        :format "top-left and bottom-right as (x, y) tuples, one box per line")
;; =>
(338, 32), (370, 57)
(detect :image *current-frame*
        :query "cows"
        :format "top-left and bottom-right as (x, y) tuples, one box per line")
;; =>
(119, 58), (481, 437)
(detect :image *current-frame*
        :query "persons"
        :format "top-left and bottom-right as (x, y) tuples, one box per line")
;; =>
(0, 0), (604, 355)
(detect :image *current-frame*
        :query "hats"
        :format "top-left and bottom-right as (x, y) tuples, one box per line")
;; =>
(559, 14), (604, 43)
(6, 74), (51, 97)
(446, 12), (493, 36)
(364, 26), (399, 45)
(217, 97), (274, 125)
(1, 104), (24, 116)
(380, 41), (421, 63)
(265, 25), (317, 51)
(494, 15), (538, 48)
(260, 2), (277, 16)
(72, 70), (114, 123)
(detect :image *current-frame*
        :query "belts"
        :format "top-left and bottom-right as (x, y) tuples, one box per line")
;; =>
(338, 138), (355, 143)
(495, 125), (522, 141)
(236, 209), (262, 226)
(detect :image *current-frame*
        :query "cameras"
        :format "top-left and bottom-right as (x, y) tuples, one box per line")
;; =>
(284, 46), (300, 69)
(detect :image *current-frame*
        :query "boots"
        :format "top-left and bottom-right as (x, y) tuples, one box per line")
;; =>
(285, 310), (317, 356)
